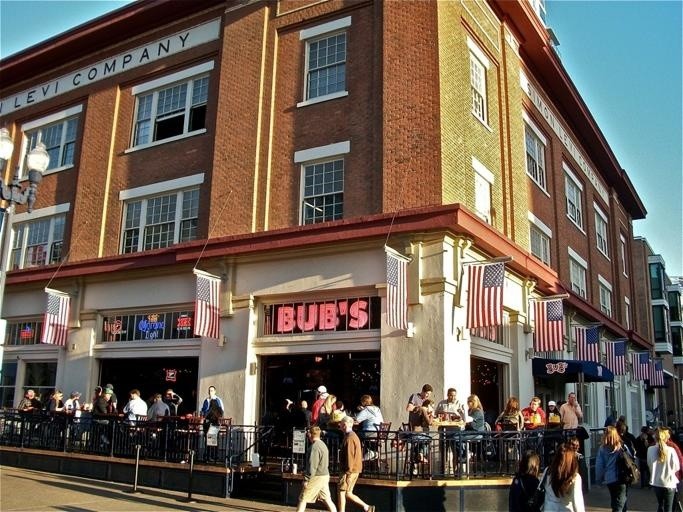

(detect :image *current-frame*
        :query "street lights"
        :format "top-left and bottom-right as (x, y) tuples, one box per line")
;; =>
(0, 126), (49, 327)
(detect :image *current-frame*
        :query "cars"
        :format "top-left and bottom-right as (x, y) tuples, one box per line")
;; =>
(671, 427), (682, 446)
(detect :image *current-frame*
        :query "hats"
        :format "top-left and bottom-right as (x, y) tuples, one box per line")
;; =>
(547, 401), (555, 406)
(317, 386), (326, 393)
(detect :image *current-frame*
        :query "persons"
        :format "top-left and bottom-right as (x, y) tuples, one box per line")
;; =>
(17, 383), (224, 465)
(509, 454), (540, 512)
(594, 411), (683, 512)
(537, 438), (585, 512)
(296, 426), (337, 512)
(286, 384), (583, 479)
(337, 415), (376, 512)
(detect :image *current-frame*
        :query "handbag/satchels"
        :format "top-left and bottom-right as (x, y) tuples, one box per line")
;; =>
(615, 450), (640, 485)
(527, 489), (544, 512)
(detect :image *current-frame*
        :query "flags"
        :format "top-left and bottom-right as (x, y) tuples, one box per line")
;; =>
(466, 262), (505, 328)
(533, 298), (564, 352)
(194, 274), (222, 340)
(575, 326), (665, 386)
(40, 292), (71, 347)
(386, 251), (408, 331)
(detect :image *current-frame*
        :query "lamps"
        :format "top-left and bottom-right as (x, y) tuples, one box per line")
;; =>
(523, 347), (535, 362)
(215, 333), (228, 348)
(455, 324), (468, 343)
(404, 320), (417, 340)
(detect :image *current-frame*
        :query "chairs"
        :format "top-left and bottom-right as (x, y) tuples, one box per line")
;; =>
(0, 398), (585, 478)
